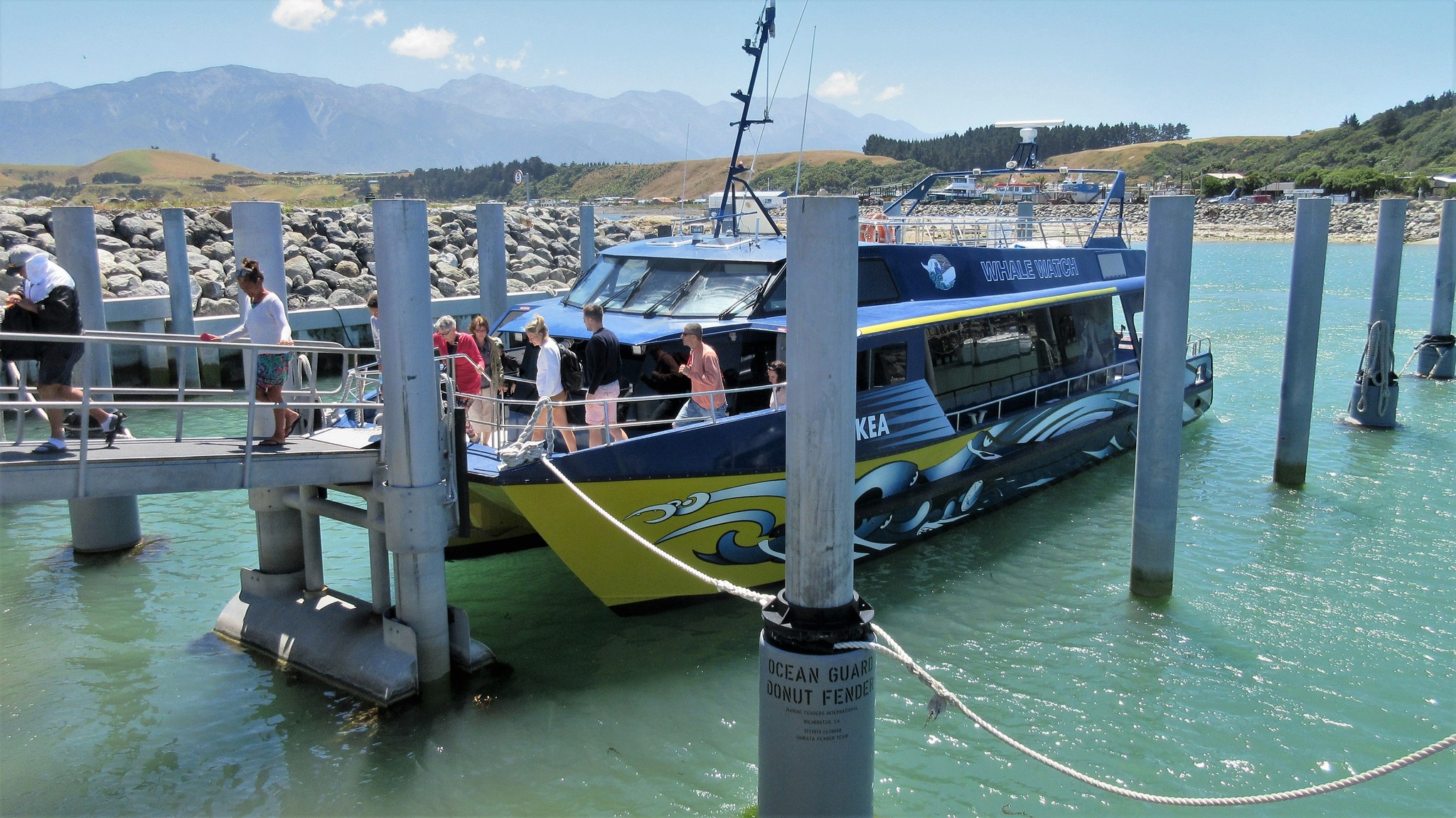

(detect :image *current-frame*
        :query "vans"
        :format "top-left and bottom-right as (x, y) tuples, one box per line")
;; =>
(1321, 194), (1348, 204)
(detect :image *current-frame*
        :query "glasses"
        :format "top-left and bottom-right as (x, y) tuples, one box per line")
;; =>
(235, 269), (254, 276)
(437, 330), (450, 336)
(683, 332), (697, 337)
(766, 363), (779, 369)
(474, 329), (488, 333)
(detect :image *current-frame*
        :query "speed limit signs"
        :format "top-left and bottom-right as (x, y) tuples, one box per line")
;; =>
(514, 169), (523, 185)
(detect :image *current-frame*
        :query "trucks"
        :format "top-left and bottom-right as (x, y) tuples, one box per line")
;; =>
(1277, 189), (1324, 205)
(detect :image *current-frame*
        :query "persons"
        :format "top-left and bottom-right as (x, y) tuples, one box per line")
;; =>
(199, 258), (300, 445)
(521, 314), (578, 453)
(367, 294), (384, 400)
(765, 361), (787, 409)
(468, 315), (521, 451)
(639, 341), (684, 428)
(669, 322), (728, 429)
(433, 315), (486, 445)
(582, 304), (629, 447)
(3, 244), (119, 451)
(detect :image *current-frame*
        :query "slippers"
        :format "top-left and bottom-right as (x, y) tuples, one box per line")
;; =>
(284, 413), (301, 438)
(32, 442), (67, 454)
(469, 436), (481, 444)
(258, 439), (286, 446)
(104, 413), (123, 448)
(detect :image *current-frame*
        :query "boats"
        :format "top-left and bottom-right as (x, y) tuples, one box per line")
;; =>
(326, 0), (1216, 616)
(853, 165), (1140, 205)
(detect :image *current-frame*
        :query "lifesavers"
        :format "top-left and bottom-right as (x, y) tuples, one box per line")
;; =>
(860, 213), (895, 244)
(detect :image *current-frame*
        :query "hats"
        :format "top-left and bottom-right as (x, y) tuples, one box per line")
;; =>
(6, 247), (36, 276)
(494, 337), (505, 346)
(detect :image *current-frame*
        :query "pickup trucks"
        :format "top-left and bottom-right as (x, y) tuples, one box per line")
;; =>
(1206, 188), (1272, 204)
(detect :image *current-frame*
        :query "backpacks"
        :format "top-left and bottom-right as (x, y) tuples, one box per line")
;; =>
(550, 337), (585, 392)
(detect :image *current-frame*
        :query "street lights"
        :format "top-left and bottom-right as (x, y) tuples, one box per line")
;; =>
(1177, 168), (1183, 195)
